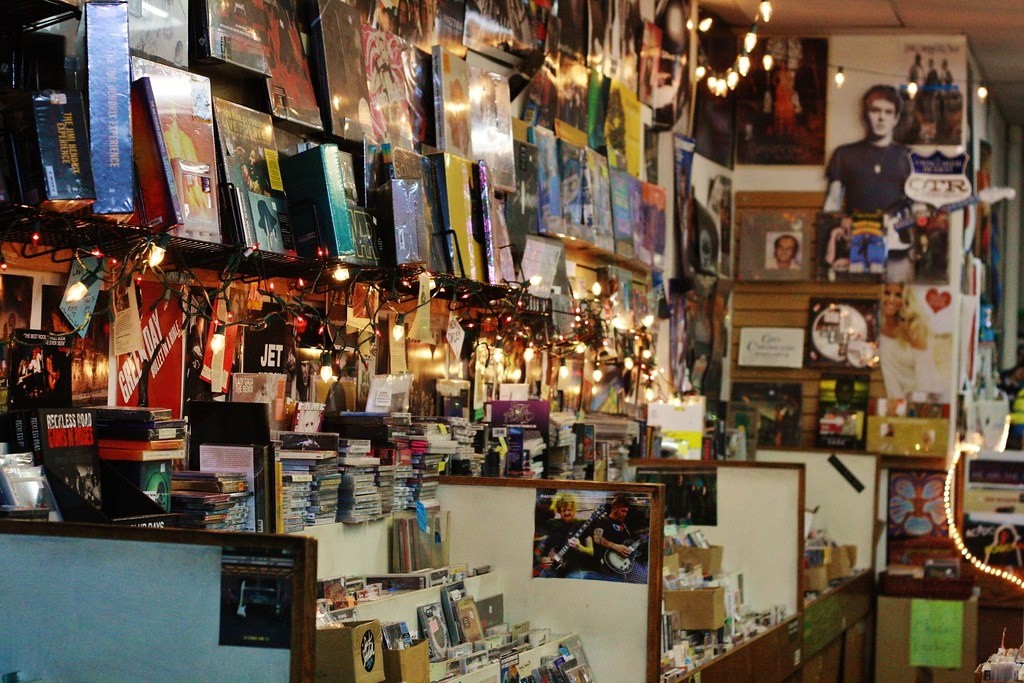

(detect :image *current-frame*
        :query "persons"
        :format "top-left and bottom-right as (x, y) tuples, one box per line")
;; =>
(823, 377), (859, 439)
(996, 361), (1024, 412)
(768, 234), (803, 275)
(898, 55), (953, 84)
(821, 84), (929, 279)
(538, 492), (648, 582)
(879, 280), (942, 416)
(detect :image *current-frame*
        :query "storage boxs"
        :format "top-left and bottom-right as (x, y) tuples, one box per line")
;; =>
(805, 566), (828, 591)
(666, 586), (724, 630)
(315, 618), (386, 683)
(675, 544), (723, 574)
(875, 588), (980, 683)
(866, 415), (952, 458)
(383, 638), (430, 683)
(828, 546), (851, 579)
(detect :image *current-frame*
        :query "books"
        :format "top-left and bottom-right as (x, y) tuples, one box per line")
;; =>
(0, 403), (284, 537)
(0, 0), (703, 290)
(885, 556), (961, 581)
(976, 340), (997, 387)
(960, 254), (994, 393)
(313, 501), (599, 683)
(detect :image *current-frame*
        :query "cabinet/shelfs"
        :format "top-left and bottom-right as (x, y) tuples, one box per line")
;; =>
(0, 443), (882, 683)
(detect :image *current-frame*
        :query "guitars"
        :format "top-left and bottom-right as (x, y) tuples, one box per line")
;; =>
(604, 533), (649, 574)
(539, 503), (608, 578)
(830, 184), (1017, 275)
